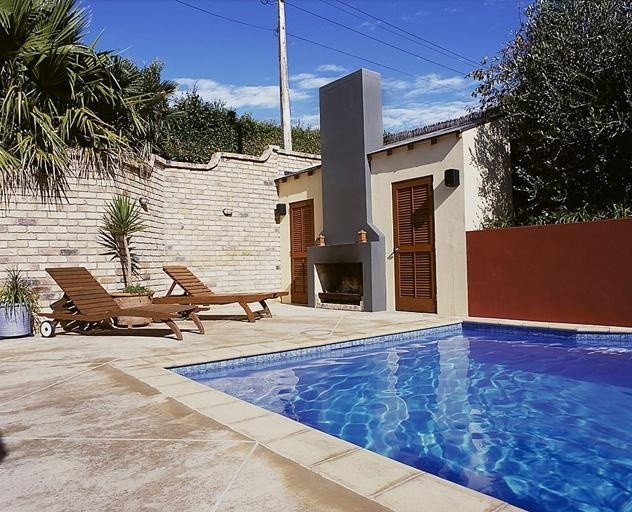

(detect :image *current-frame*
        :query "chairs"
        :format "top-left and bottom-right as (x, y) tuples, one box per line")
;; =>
(151, 265), (290, 323)
(37, 266), (206, 341)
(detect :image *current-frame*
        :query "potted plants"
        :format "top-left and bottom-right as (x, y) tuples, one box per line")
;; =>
(0, 268), (39, 338)
(95, 188), (156, 329)
